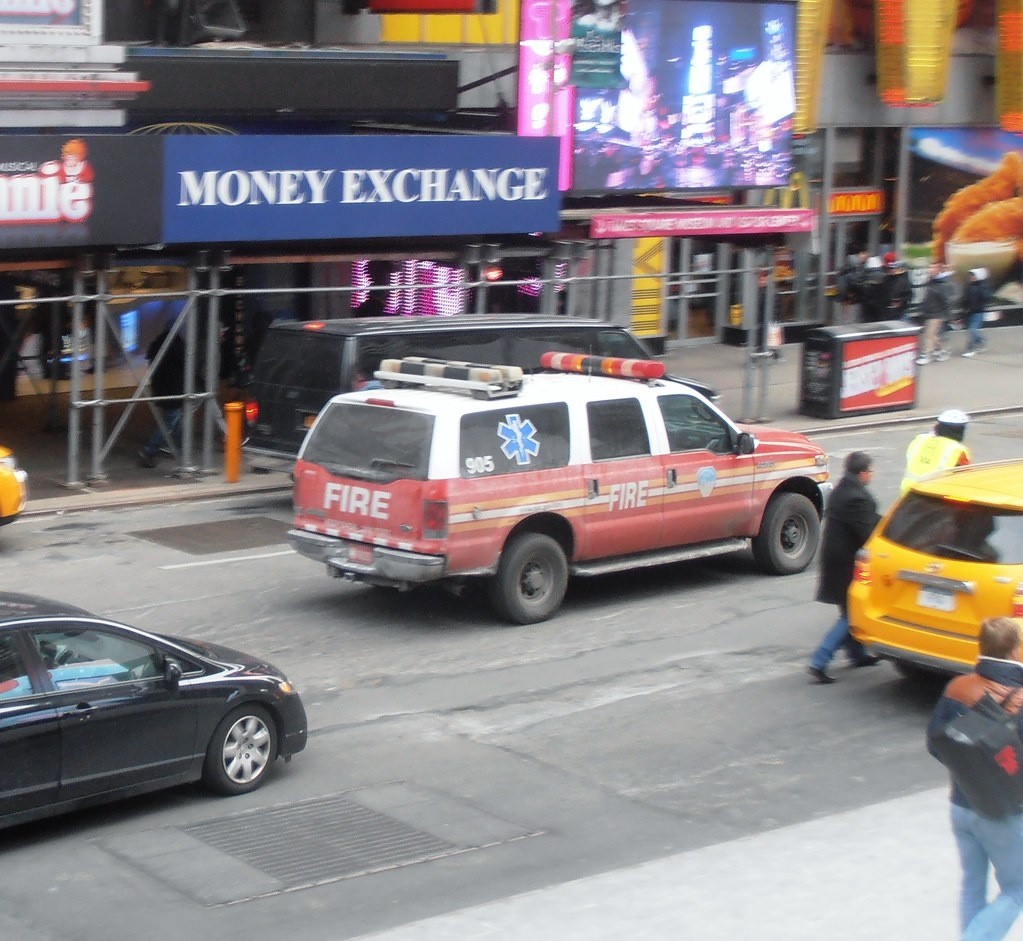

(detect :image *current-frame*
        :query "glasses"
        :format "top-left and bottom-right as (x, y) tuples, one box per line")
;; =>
(861, 468), (875, 475)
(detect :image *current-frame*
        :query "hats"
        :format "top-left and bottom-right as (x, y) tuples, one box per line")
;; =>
(969, 266), (986, 280)
(864, 255), (883, 269)
(884, 253), (896, 265)
(937, 409), (971, 424)
(932, 267), (954, 279)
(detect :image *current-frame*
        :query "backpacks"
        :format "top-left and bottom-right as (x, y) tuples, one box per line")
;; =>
(937, 684), (1023, 821)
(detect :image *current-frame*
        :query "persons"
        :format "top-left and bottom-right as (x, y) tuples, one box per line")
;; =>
(900, 408), (971, 499)
(962, 267), (988, 358)
(842, 248), (913, 321)
(915, 261), (952, 365)
(926, 617), (1023, 941)
(804, 450), (879, 683)
(136, 318), (205, 468)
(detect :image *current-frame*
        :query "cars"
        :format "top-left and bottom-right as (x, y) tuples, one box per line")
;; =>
(1, 445), (30, 527)
(0, 588), (309, 831)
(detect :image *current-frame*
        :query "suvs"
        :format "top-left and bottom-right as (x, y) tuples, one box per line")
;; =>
(846, 457), (1023, 682)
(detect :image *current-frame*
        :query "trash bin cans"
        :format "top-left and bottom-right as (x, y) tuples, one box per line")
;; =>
(798, 320), (927, 420)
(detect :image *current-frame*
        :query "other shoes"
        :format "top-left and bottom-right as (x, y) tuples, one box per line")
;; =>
(962, 337), (987, 357)
(854, 655), (881, 667)
(809, 667), (836, 683)
(914, 348), (953, 349)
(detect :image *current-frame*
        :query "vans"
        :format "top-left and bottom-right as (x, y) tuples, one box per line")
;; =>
(241, 311), (727, 485)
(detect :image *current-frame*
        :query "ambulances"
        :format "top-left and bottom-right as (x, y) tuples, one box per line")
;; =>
(288, 352), (834, 624)
